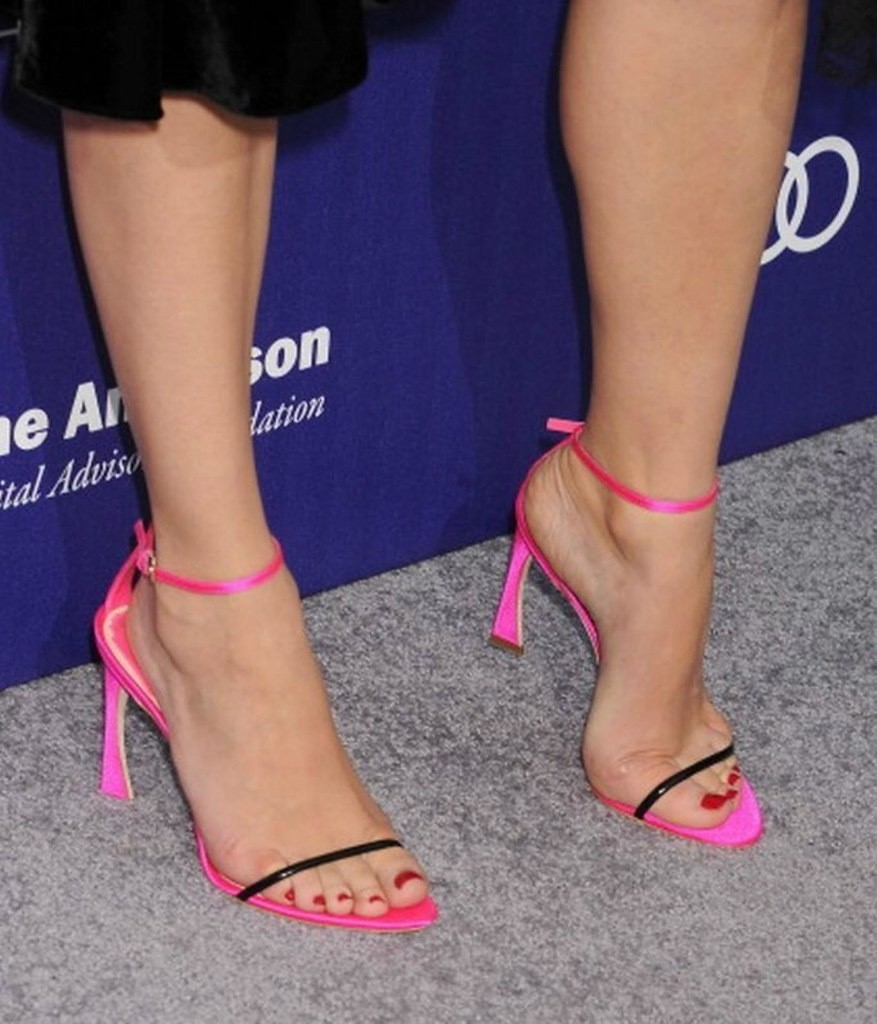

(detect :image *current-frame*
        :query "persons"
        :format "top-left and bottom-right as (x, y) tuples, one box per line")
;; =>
(0, 0), (809, 933)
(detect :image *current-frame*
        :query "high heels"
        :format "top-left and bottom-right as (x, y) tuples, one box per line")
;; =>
(487, 417), (762, 848)
(92, 519), (440, 932)
